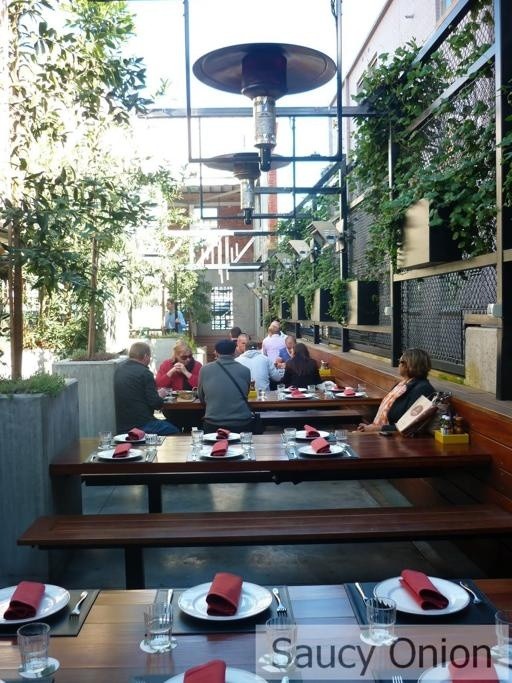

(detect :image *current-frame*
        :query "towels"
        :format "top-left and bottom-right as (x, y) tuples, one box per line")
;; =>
(288, 385), (356, 398)
(181, 656), (226, 683)
(211, 423), (331, 458)
(204, 570), (244, 617)
(443, 655), (498, 682)
(5, 579), (43, 619)
(394, 565), (450, 611)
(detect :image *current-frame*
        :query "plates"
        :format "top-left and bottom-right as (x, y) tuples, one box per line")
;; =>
(200, 445), (244, 459)
(203, 427), (241, 441)
(293, 430), (329, 440)
(96, 446), (143, 460)
(418, 658), (511, 682)
(171, 389), (194, 395)
(176, 579), (273, 622)
(372, 575), (473, 617)
(284, 387), (368, 398)
(163, 668), (272, 682)
(1, 582), (73, 625)
(298, 445), (345, 456)
(114, 430), (153, 442)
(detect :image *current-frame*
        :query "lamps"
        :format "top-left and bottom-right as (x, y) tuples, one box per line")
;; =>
(192, 38), (341, 172)
(208, 149), (291, 226)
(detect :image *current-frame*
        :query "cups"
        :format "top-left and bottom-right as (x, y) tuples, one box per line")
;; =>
(191, 430), (203, 447)
(283, 427), (296, 445)
(240, 431), (252, 449)
(334, 428), (348, 442)
(494, 607), (512, 667)
(257, 388), (266, 400)
(365, 598), (398, 644)
(145, 434), (157, 445)
(142, 601), (176, 651)
(17, 622), (51, 675)
(264, 616), (299, 672)
(276, 384), (285, 393)
(99, 430), (112, 450)
(165, 387), (172, 398)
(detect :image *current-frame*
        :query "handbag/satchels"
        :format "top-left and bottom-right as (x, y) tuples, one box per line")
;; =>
(395, 391), (439, 436)
(251, 412), (265, 434)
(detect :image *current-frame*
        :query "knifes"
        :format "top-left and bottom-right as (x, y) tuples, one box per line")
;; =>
(145, 448), (158, 463)
(355, 582), (371, 607)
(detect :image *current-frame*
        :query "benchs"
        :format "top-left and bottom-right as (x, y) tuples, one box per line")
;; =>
(17, 507), (510, 585)
(250, 409), (363, 430)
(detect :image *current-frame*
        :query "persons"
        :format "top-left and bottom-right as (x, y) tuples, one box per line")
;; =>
(163, 297), (189, 333)
(114, 341), (182, 435)
(155, 318), (323, 432)
(354, 347), (440, 432)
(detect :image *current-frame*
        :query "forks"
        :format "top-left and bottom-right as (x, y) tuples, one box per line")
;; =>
(459, 579), (486, 606)
(90, 453), (97, 462)
(67, 589), (89, 622)
(245, 451), (252, 458)
(272, 587), (288, 618)
(159, 588), (175, 626)
(289, 448), (297, 458)
(390, 674), (403, 682)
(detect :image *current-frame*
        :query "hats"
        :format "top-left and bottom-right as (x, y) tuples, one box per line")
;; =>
(245, 342), (257, 350)
(215, 339), (236, 355)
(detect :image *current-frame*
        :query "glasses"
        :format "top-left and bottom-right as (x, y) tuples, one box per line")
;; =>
(178, 354), (193, 361)
(397, 359), (403, 364)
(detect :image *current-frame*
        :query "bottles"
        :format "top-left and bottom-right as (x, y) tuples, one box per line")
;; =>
(320, 359), (329, 370)
(441, 415), (463, 435)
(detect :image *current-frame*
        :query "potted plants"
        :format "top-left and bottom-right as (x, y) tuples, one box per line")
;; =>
(0, 1), (167, 581)
